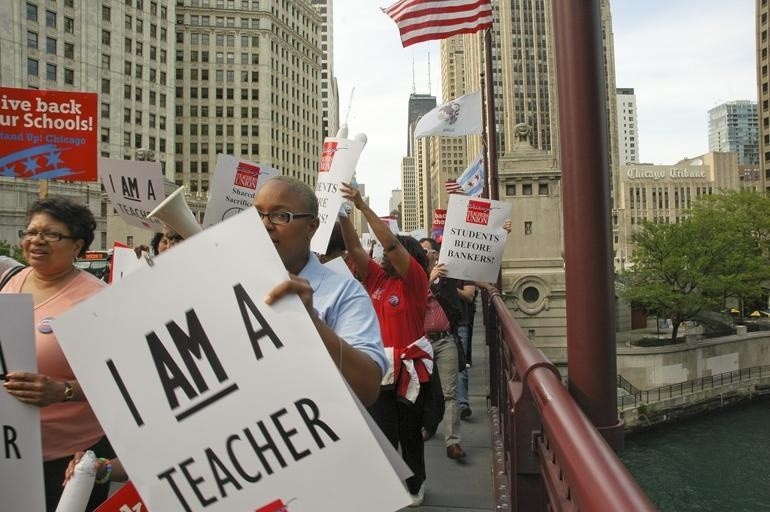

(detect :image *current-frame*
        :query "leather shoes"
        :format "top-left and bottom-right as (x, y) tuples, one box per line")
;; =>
(422, 430), (434, 441)
(447, 444), (466, 458)
(460, 407), (472, 419)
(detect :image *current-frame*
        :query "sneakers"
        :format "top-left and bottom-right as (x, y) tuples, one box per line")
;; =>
(409, 485), (424, 506)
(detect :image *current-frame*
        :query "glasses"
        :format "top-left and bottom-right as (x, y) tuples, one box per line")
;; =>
(258, 211), (314, 224)
(18, 230), (74, 242)
(423, 248), (436, 256)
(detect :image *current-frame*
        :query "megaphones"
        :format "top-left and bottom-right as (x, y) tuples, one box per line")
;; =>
(147, 185), (201, 239)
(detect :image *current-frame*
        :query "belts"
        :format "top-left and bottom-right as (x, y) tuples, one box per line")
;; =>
(428, 330), (453, 341)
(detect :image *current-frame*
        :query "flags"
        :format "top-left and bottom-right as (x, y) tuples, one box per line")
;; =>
(413, 88), (484, 138)
(445, 146), (485, 197)
(378, 0), (495, 47)
